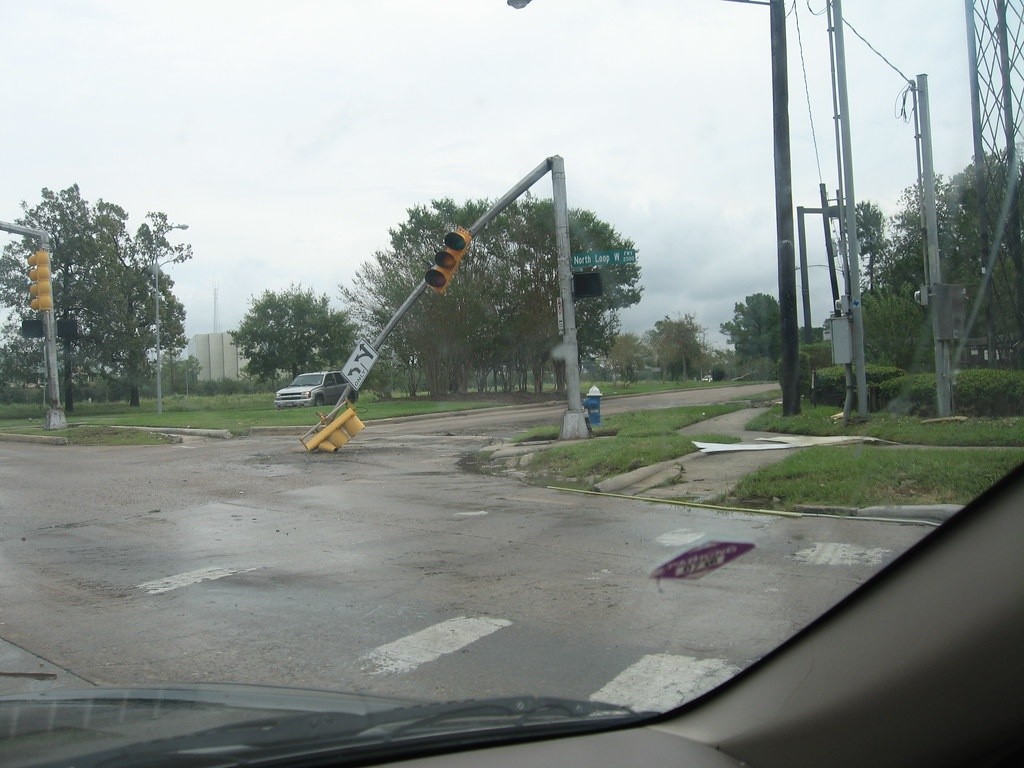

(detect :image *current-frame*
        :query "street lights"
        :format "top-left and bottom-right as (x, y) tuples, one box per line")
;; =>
(156, 223), (190, 413)
(505, 0), (800, 418)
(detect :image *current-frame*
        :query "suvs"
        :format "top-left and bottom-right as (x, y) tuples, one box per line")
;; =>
(272, 370), (359, 410)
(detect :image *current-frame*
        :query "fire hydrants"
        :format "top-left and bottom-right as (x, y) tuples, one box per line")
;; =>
(583, 386), (603, 426)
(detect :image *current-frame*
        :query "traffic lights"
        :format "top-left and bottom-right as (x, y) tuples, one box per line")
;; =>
(29, 252), (54, 312)
(304, 406), (366, 454)
(56, 318), (79, 339)
(423, 227), (474, 294)
(20, 320), (45, 340)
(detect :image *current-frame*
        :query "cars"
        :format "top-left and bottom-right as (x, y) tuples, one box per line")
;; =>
(702, 375), (713, 383)
